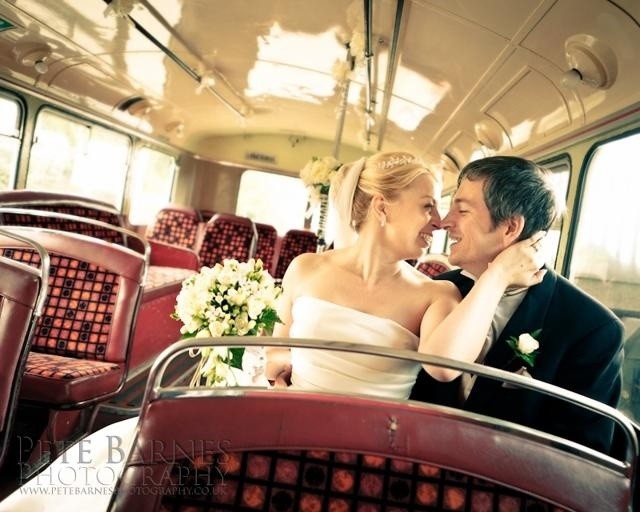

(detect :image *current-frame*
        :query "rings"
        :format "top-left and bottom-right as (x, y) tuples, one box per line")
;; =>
(530, 243), (541, 252)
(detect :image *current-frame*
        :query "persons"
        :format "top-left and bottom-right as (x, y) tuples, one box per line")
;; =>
(273, 155), (627, 457)
(249, 150), (553, 401)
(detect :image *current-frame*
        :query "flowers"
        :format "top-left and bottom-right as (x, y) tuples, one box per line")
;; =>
(298, 156), (343, 194)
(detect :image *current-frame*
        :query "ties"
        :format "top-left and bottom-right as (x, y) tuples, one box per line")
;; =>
(470, 287), (528, 378)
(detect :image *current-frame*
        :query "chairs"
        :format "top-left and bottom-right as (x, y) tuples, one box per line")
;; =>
(1, 189), (639, 512)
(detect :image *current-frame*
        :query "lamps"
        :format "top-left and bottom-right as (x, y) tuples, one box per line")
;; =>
(163, 120), (185, 139)
(21, 47), (53, 75)
(440, 154), (458, 173)
(471, 119), (503, 151)
(559, 44), (606, 93)
